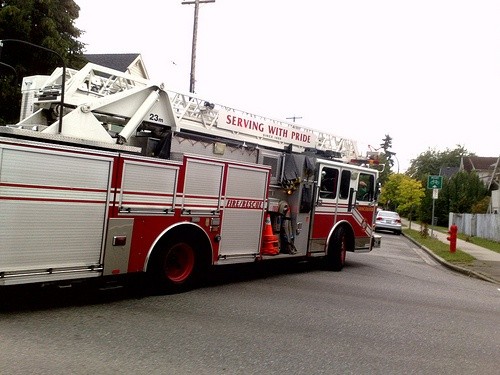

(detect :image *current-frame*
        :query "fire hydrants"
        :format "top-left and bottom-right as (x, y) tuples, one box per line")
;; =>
(447, 224), (458, 254)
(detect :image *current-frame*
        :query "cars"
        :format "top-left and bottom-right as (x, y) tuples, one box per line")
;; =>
(374, 211), (402, 235)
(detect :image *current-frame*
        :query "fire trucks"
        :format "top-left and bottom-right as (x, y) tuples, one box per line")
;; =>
(0, 39), (381, 294)
